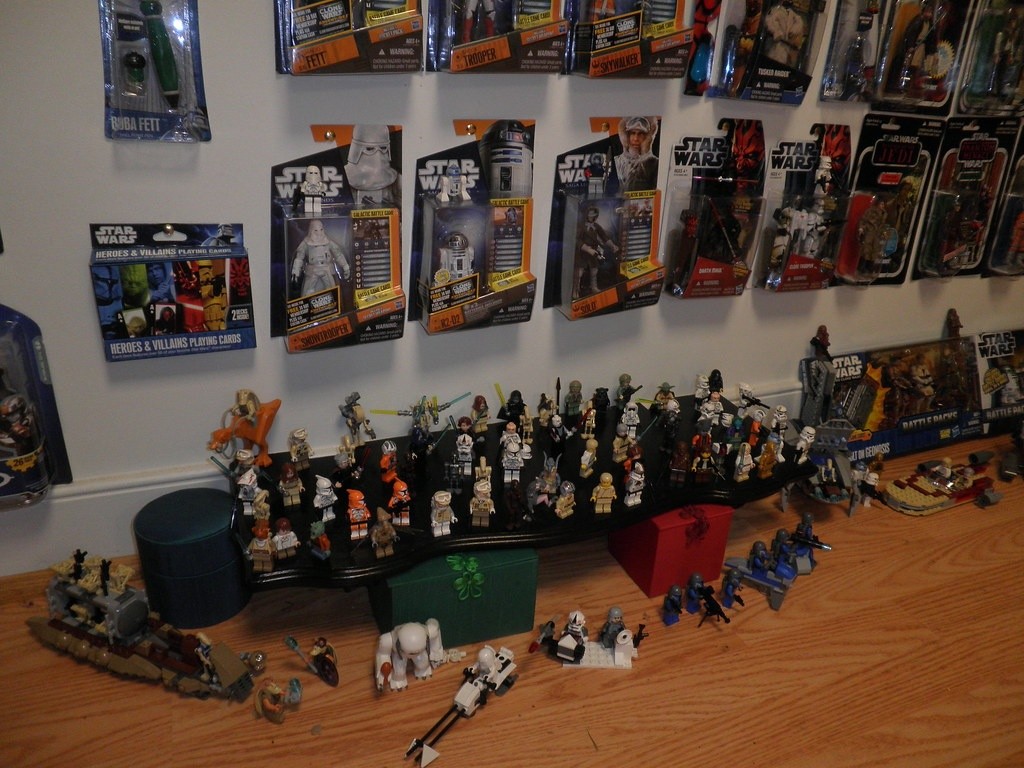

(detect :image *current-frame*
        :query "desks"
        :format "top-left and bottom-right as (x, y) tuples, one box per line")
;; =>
(1, 429), (1024, 768)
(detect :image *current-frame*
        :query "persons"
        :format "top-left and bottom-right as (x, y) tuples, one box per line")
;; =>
(984, 16), (1018, 99)
(911, 353), (934, 414)
(460, 0), (495, 45)
(803, 199), (825, 257)
(1003, 211), (1024, 268)
(469, 645), (496, 692)
(896, 0), (939, 91)
(765, 1), (803, 65)
(781, 196), (809, 258)
(856, 198), (888, 282)
(612, 117), (660, 193)
(573, 206), (619, 301)
(889, 357), (912, 426)
(231, 371), (816, 647)
(290, 219), (350, 301)
